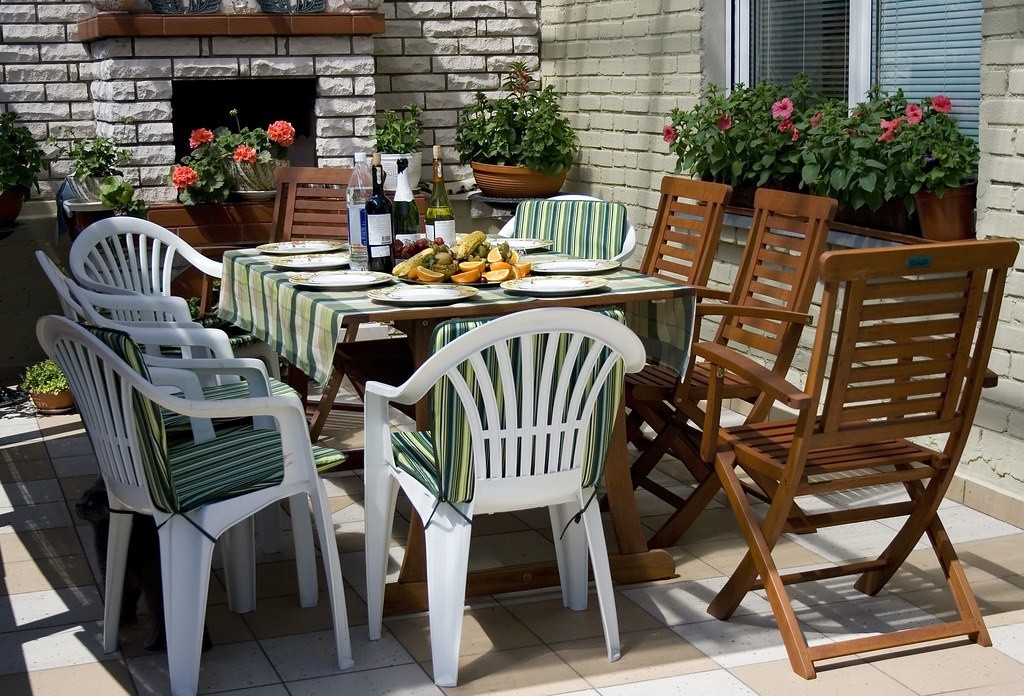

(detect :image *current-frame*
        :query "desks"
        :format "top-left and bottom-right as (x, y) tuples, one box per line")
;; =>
(217, 231), (698, 618)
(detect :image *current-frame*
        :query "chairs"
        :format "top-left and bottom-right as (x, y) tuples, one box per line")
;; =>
(36, 167), (1020, 696)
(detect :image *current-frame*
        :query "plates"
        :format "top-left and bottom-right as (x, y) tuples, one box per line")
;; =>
(394, 275), (501, 287)
(256, 240), (345, 257)
(289, 269), (393, 290)
(484, 238), (555, 251)
(500, 276), (609, 295)
(530, 259), (621, 274)
(267, 253), (350, 271)
(367, 284), (480, 304)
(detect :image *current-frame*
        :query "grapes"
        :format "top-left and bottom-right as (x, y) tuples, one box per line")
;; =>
(395, 237), (444, 259)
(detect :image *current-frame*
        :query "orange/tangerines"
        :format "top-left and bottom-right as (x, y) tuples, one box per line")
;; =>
(408, 247), (532, 284)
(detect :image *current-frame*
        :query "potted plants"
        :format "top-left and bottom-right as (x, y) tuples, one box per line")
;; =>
(367, 103), (425, 189)
(18, 358), (75, 410)
(0, 110), (50, 225)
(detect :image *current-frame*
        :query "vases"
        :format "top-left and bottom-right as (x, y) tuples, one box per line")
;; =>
(470, 161), (566, 197)
(225, 160), (290, 191)
(66, 175), (124, 203)
(912, 181), (977, 243)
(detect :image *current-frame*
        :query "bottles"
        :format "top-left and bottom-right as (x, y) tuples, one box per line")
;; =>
(365, 153), (395, 276)
(392, 158), (420, 265)
(346, 152), (373, 271)
(424, 145), (456, 248)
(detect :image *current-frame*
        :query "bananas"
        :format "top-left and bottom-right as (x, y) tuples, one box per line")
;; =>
(457, 230), (487, 260)
(392, 248), (434, 277)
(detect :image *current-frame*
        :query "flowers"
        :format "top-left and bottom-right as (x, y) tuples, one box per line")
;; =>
(663, 72), (982, 220)
(454, 61), (579, 177)
(167, 108), (296, 206)
(44, 126), (149, 267)
